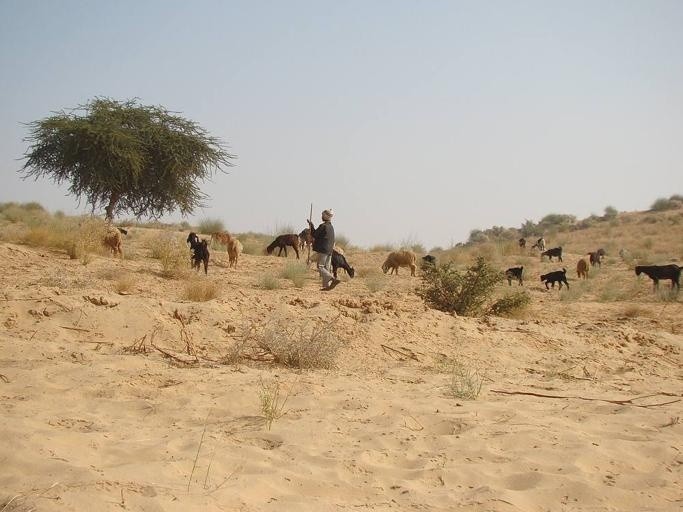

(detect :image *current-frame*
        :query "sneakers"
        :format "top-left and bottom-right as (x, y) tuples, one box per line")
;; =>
(330, 279), (340, 289)
(320, 287), (330, 291)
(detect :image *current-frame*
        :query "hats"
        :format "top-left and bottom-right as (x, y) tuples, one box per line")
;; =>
(322, 209), (333, 220)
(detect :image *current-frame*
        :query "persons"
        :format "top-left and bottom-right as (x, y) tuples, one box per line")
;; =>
(306, 208), (341, 291)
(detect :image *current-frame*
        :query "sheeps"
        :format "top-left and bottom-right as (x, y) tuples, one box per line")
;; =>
(382, 251), (416, 277)
(505, 266), (523, 286)
(306, 246), (347, 274)
(267, 234), (300, 259)
(331, 250), (354, 279)
(635, 264), (683, 295)
(422, 255), (436, 263)
(299, 228), (315, 251)
(105, 227), (127, 259)
(187, 231), (244, 274)
(518, 237), (606, 291)
